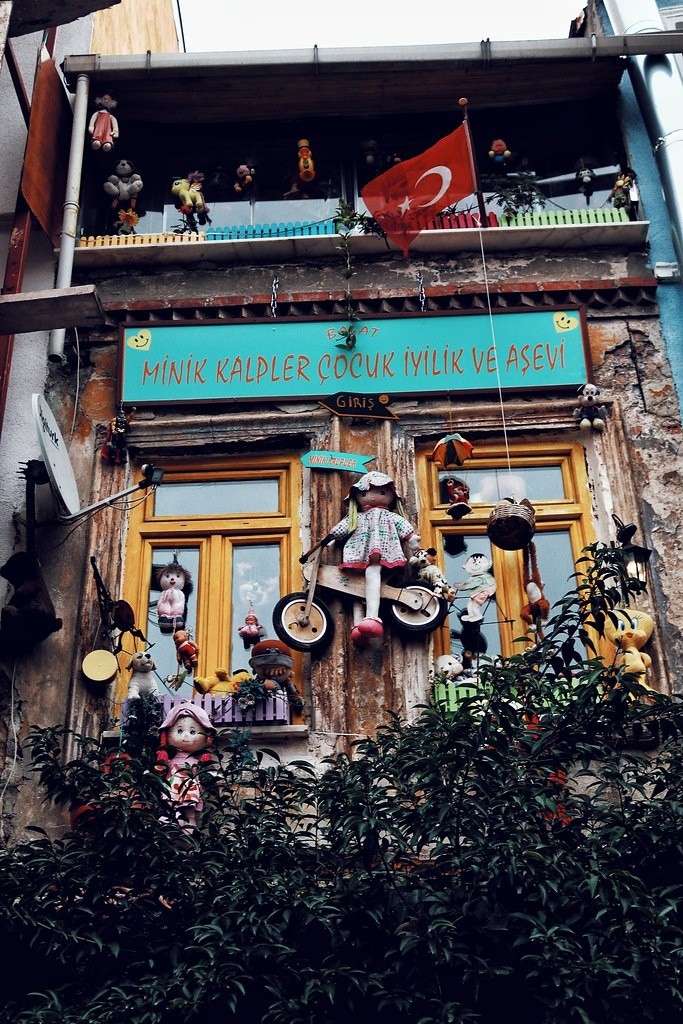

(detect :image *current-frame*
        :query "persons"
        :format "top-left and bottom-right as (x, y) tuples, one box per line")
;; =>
(99, 406), (136, 466)
(173, 630), (199, 668)
(157, 562), (191, 629)
(238, 614), (263, 651)
(155, 702), (218, 834)
(321, 472), (497, 646)
(248, 640), (304, 712)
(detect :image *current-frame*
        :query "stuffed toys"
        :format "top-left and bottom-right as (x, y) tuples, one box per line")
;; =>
(433, 653), (481, 689)
(124, 651), (159, 702)
(603, 609), (660, 703)
(520, 541), (550, 641)
(0, 552), (62, 661)
(572, 383), (609, 430)
(87, 91), (641, 218)
(193, 668), (253, 698)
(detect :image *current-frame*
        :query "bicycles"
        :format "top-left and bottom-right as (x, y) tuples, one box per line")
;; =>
(271, 533), (450, 653)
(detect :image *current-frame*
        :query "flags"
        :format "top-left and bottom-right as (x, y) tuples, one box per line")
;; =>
(359, 119), (478, 253)
